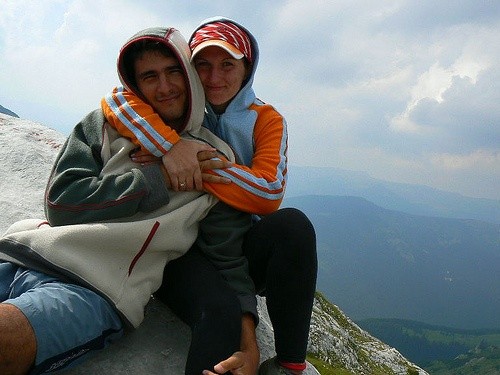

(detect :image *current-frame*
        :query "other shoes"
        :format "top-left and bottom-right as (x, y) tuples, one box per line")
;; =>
(258, 353), (301, 375)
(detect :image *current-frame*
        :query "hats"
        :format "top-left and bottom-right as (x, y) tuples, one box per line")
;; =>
(190, 20), (252, 65)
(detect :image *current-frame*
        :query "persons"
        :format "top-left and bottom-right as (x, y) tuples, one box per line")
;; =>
(0, 16), (321, 374)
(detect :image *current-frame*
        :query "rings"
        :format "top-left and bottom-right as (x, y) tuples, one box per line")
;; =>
(179, 182), (186, 187)
